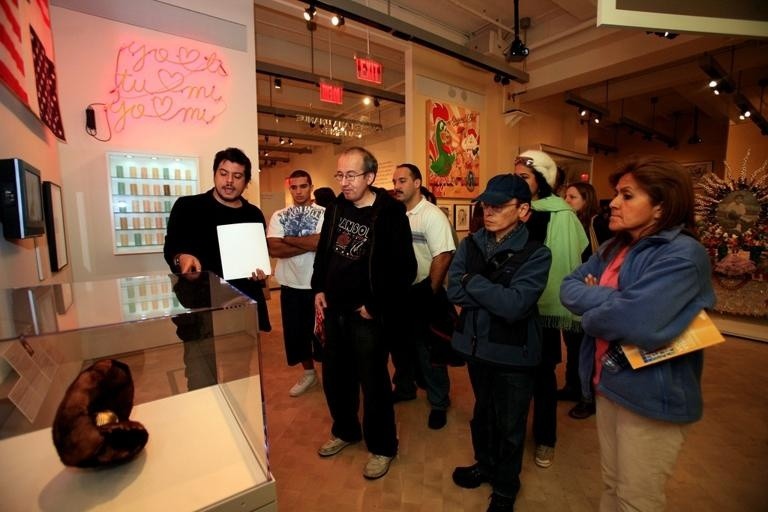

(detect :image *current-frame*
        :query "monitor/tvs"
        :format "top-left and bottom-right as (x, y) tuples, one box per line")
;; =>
(11, 285), (58, 335)
(55, 284), (74, 314)
(0, 158), (45, 240)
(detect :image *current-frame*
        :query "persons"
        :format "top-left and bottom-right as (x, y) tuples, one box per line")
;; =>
(311, 146), (418, 478)
(452, 174), (553, 511)
(515, 150), (610, 466)
(391, 164), (458, 428)
(266, 170), (337, 396)
(558, 156), (718, 511)
(163, 147), (272, 392)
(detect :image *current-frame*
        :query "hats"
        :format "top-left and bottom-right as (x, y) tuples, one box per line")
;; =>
(515, 147), (558, 190)
(471, 173), (531, 205)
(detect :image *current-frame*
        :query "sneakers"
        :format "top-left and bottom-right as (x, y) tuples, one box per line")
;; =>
(534, 444), (555, 468)
(362, 452), (395, 479)
(555, 388), (596, 419)
(392, 388), (416, 403)
(291, 369), (318, 397)
(318, 435), (352, 456)
(428, 407), (446, 429)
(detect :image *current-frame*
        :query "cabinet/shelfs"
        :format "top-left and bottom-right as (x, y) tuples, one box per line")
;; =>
(118, 277), (193, 320)
(106, 152), (200, 254)
(519, 143), (594, 200)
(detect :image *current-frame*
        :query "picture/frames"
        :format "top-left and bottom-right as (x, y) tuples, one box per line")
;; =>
(437, 204), (453, 228)
(471, 204), (476, 219)
(679, 160), (714, 183)
(455, 204), (471, 232)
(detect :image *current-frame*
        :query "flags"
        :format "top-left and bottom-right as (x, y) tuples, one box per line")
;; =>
(0, 0), (68, 143)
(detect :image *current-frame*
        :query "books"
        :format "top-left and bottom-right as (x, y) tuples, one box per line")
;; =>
(619, 308), (727, 370)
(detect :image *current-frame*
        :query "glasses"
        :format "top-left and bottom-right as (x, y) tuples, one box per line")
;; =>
(514, 156), (533, 167)
(333, 173), (365, 181)
(481, 201), (519, 212)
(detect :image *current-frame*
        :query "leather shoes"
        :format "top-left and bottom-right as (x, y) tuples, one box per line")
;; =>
(453, 462), (516, 511)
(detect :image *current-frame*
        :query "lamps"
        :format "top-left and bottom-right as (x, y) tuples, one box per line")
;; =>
(295, 0), (530, 87)
(566, 81), (610, 124)
(256, 60), (405, 171)
(734, 66), (767, 136)
(700, 51), (734, 97)
(620, 98), (656, 141)
